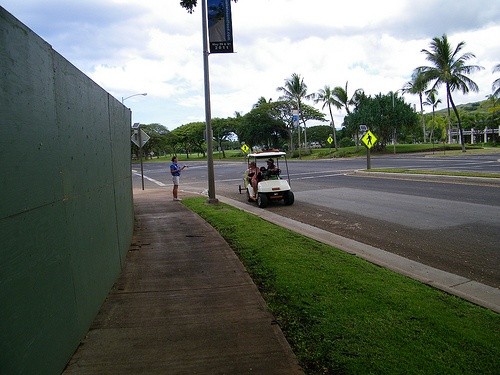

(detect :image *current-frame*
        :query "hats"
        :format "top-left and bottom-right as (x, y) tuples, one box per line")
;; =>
(266, 159), (274, 163)
(249, 161), (255, 166)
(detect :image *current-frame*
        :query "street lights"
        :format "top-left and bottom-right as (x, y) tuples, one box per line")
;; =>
(121, 93), (148, 104)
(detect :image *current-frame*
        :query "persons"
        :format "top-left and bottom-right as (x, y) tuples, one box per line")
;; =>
(269, 165), (280, 180)
(245, 162), (260, 197)
(267, 159), (275, 169)
(257, 167), (270, 193)
(169, 156), (189, 200)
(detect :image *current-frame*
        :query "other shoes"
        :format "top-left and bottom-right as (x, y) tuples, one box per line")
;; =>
(175, 198), (182, 201)
